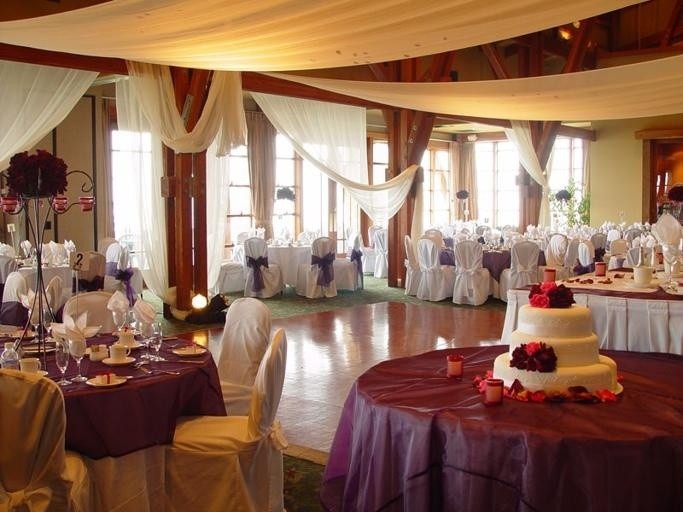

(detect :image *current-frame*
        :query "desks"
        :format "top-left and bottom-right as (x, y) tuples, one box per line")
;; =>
(3, 331), (228, 511)
(264, 238), (312, 299)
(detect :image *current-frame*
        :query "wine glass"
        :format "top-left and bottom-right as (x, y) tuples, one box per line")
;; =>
(112, 306), (166, 364)
(27, 307), (53, 345)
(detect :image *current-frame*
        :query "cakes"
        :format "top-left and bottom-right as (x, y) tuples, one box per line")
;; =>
(493, 282), (618, 401)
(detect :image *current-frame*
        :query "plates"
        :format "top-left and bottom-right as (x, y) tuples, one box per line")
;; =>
(0, 325), (207, 388)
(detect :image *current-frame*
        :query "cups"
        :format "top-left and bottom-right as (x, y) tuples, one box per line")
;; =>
(483, 379), (505, 405)
(633, 265), (654, 285)
(594, 262), (607, 276)
(445, 354), (464, 378)
(541, 268), (556, 283)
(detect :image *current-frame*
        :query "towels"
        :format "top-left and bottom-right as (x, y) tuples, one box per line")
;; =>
(652, 213), (682, 244)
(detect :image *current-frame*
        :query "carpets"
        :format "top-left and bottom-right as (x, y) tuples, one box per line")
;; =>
(283, 453), (326, 512)
(141, 272), (506, 337)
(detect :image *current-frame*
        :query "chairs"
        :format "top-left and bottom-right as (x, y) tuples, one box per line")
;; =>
(537, 235), (569, 281)
(0, 367), (92, 511)
(361, 225), (382, 276)
(299, 237), (338, 298)
(374, 228), (388, 279)
(417, 239), (456, 302)
(452, 240), (490, 306)
(333, 232), (363, 292)
(576, 241), (597, 273)
(500, 240), (540, 303)
(1, 223), (287, 336)
(298, 229), (317, 246)
(217, 296), (272, 414)
(345, 227), (375, 275)
(625, 248), (653, 266)
(404, 235), (419, 296)
(602, 239), (626, 266)
(419, 221), (656, 254)
(167, 326), (287, 510)
